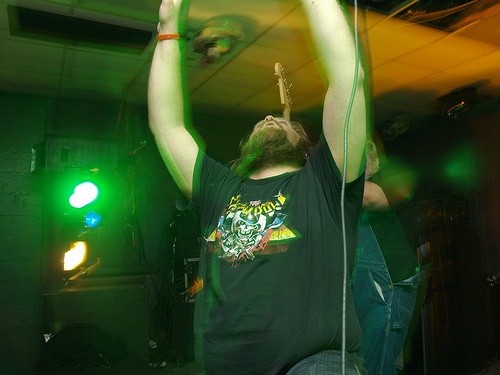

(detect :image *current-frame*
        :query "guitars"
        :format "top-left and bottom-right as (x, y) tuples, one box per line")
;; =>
(274, 61), (293, 121)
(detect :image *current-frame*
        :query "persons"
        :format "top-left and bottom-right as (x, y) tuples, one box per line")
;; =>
(148, 0), (367, 375)
(381, 172), (431, 375)
(351, 141), (422, 375)
(155, 192), (201, 369)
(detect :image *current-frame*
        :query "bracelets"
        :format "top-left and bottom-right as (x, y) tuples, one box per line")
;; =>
(156, 33), (179, 42)
